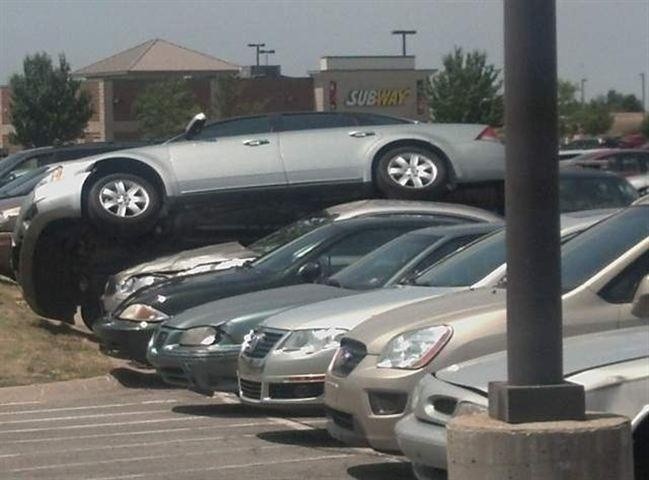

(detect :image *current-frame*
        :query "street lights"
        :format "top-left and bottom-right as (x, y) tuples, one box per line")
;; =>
(391, 29), (417, 55)
(259, 49), (275, 66)
(638, 72), (646, 111)
(580, 77), (588, 102)
(246, 42), (265, 65)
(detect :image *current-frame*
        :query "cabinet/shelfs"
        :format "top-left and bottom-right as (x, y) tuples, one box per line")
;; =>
(346, 243), (400, 276)
